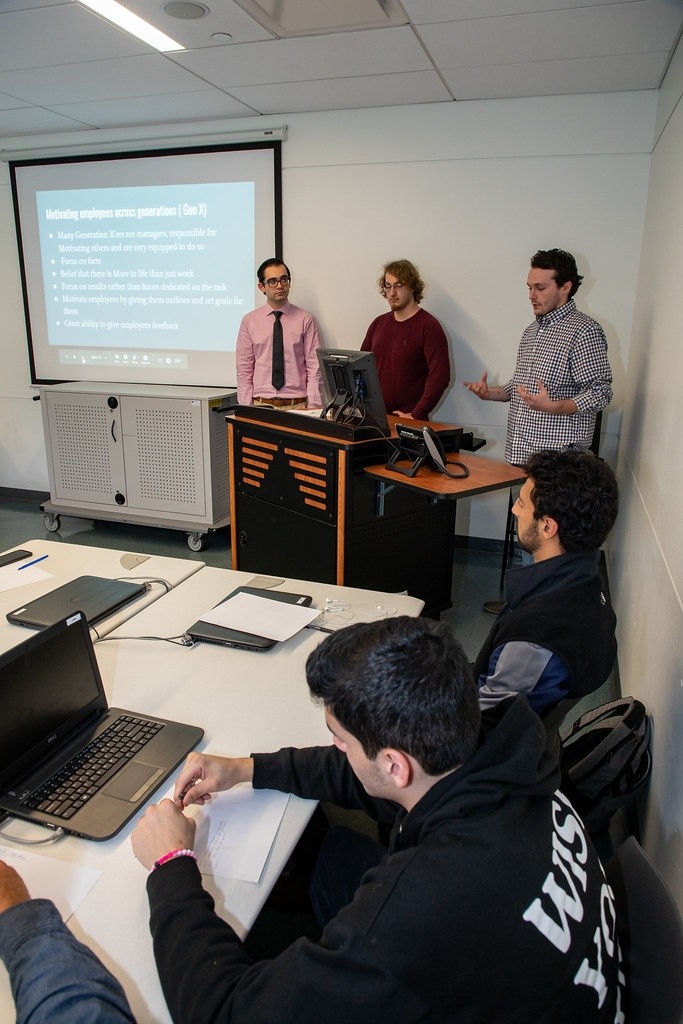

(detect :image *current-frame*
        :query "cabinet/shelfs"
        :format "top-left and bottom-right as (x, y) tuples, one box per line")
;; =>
(38, 381), (239, 550)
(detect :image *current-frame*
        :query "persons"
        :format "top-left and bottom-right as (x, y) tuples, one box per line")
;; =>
(133, 616), (634, 1024)
(235, 258), (324, 410)
(0, 860), (138, 1024)
(321, 450), (619, 843)
(460, 249), (612, 615)
(360, 260), (450, 419)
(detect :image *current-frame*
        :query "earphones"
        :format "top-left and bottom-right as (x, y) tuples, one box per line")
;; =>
(326, 597), (338, 603)
(325, 606), (343, 612)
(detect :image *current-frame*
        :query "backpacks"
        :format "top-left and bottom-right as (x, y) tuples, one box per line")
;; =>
(561, 695), (653, 835)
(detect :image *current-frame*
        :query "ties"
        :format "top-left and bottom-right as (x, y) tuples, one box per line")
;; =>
(271, 311), (284, 391)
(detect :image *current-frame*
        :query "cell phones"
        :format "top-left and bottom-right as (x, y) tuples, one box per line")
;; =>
(0, 550), (33, 567)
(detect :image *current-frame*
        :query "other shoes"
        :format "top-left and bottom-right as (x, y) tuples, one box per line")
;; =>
(483, 601), (509, 614)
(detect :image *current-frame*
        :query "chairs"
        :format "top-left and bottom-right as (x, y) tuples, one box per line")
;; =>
(609, 836), (683, 1024)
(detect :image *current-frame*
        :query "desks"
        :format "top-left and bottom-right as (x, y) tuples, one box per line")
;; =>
(0, 539), (425, 1024)
(363, 451), (529, 517)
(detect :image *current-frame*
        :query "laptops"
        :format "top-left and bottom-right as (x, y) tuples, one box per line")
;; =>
(186, 586), (313, 652)
(6, 575), (147, 631)
(0, 610), (204, 843)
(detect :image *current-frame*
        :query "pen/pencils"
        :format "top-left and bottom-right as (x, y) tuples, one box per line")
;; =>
(18, 555), (49, 570)
(175, 779), (197, 805)
(306, 625), (334, 633)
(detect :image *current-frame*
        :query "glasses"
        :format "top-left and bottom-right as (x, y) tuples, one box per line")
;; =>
(383, 283), (403, 291)
(262, 277), (290, 287)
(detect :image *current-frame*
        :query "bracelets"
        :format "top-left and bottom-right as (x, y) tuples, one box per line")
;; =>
(152, 849), (197, 872)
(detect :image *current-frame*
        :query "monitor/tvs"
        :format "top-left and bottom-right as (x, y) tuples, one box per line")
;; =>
(317, 347), (393, 438)
